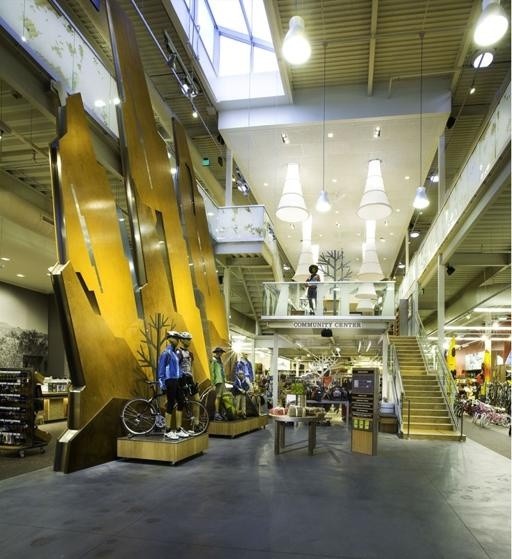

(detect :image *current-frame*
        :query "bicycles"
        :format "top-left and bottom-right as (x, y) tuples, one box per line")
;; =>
(121, 379), (211, 439)
(455, 394), (511, 428)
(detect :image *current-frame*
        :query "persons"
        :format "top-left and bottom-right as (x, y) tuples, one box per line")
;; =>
(160, 330), (253, 441)
(305, 264), (321, 316)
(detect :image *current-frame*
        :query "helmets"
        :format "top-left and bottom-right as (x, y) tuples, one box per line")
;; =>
(167, 331), (181, 339)
(180, 332), (192, 338)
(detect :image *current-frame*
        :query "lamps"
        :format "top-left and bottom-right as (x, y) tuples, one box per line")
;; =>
(166, 51), (178, 69)
(278, 0), (313, 67)
(293, 218), (315, 280)
(274, 161), (308, 221)
(358, 159), (392, 221)
(314, 38), (334, 215)
(412, 32), (431, 211)
(472, 1), (509, 47)
(357, 220), (386, 281)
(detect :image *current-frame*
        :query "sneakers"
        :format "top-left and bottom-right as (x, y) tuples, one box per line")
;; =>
(164, 430), (179, 440)
(215, 413), (223, 421)
(174, 427), (190, 437)
(240, 411), (247, 419)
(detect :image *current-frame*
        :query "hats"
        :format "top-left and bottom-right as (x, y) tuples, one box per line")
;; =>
(309, 265), (318, 273)
(212, 348), (225, 353)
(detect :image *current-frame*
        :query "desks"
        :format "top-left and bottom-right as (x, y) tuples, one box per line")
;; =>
(307, 399), (350, 426)
(270, 413), (327, 456)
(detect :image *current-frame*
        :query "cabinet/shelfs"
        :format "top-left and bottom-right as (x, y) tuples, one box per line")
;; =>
(0, 368), (52, 458)
